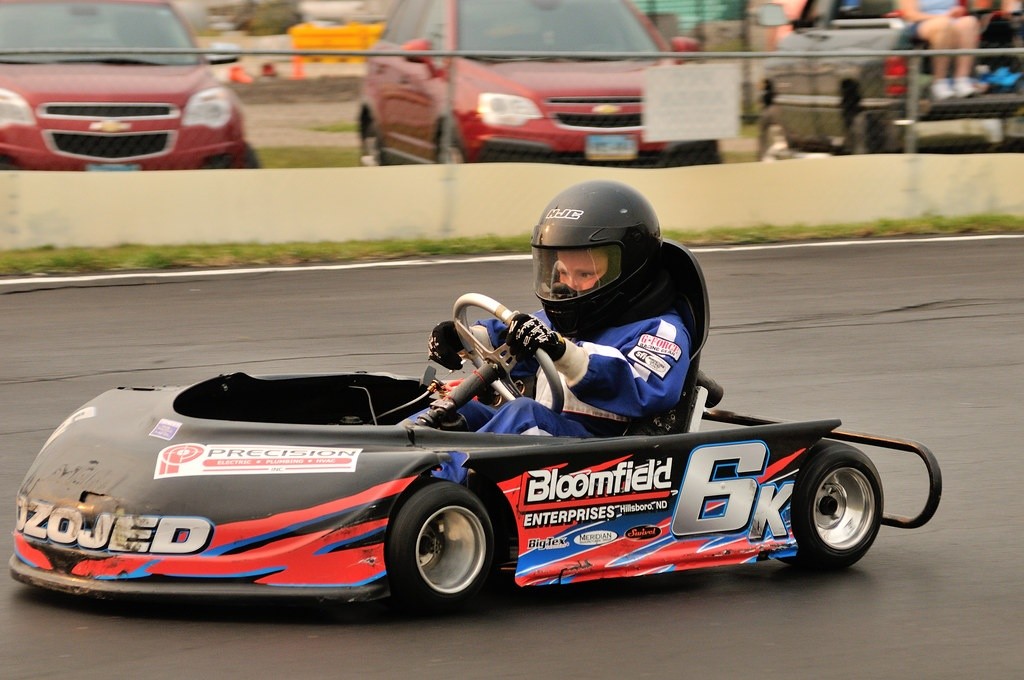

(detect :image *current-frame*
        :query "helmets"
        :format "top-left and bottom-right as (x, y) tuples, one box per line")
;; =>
(531, 180), (669, 338)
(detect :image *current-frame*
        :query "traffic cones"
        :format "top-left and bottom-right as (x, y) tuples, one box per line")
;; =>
(226, 65), (254, 82)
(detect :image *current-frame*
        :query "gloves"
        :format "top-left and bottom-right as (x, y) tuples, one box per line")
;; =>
(506, 313), (566, 362)
(429, 321), (464, 370)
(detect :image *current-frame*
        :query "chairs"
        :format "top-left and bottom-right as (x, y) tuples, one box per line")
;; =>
(636, 240), (712, 437)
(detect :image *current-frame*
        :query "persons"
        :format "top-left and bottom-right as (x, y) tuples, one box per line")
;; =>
(388, 178), (693, 441)
(830, 0), (1024, 101)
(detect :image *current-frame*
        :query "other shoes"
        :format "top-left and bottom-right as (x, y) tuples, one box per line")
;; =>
(929, 77), (977, 100)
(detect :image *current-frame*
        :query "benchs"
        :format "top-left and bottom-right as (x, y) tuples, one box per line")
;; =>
(909, 15), (1015, 81)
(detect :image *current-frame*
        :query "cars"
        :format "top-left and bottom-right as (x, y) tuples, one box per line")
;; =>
(359, 1), (720, 166)
(0, 0), (262, 174)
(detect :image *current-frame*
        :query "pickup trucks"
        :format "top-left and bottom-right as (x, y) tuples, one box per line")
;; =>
(757, 0), (1024, 155)
